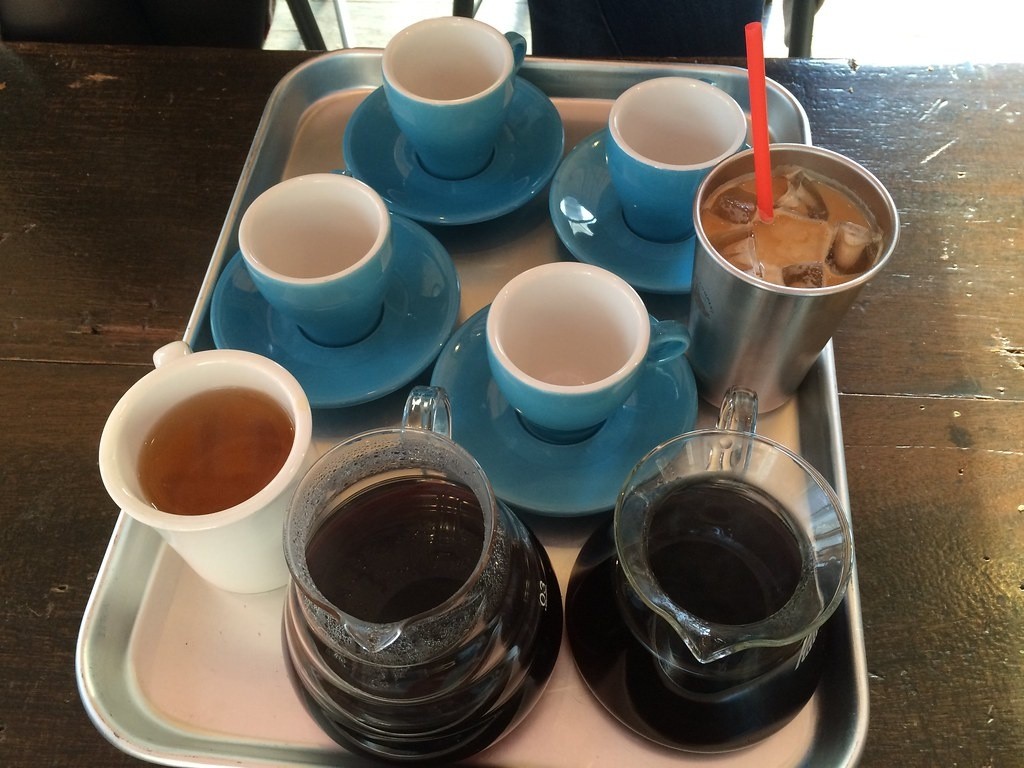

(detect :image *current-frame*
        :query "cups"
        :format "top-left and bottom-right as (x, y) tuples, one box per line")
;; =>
(485, 262), (690, 443)
(98, 341), (319, 592)
(238, 169), (392, 345)
(689, 143), (899, 414)
(602, 76), (747, 241)
(381, 16), (528, 179)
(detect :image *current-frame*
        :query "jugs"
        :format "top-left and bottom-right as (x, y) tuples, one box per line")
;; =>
(564, 388), (856, 755)
(281, 385), (565, 768)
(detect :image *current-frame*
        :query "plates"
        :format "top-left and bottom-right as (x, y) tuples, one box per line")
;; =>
(551, 126), (751, 295)
(210, 213), (462, 411)
(429, 303), (699, 517)
(343, 75), (565, 226)
